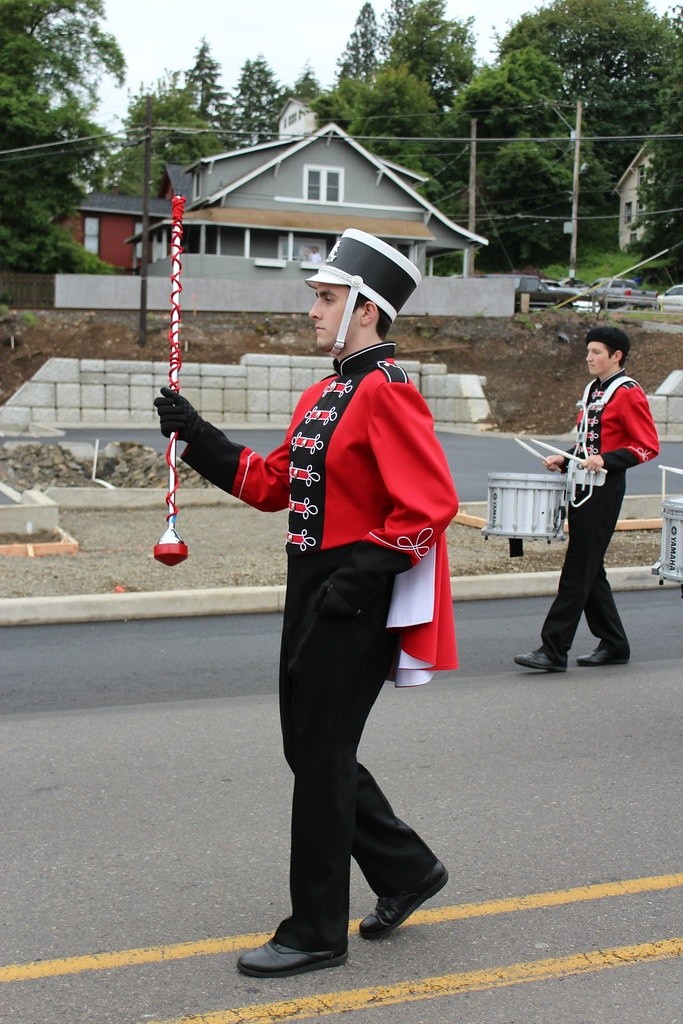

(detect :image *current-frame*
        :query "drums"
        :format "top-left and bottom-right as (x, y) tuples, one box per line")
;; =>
(650, 494), (682, 585)
(480, 474), (574, 543)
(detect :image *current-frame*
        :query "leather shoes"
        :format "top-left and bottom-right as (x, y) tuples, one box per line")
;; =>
(514, 646), (568, 673)
(236, 937), (348, 979)
(359, 860), (449, 940)
(575, 649), (630, 667)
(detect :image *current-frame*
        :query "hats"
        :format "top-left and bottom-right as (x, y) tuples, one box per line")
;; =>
(305, 227), (423, 355)
(585, 326), (631, 357)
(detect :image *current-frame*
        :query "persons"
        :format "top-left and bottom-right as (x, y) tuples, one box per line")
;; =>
(309, 246), (321, 261)
(513, 327), (660, 673)
(152, 229), (459, 978)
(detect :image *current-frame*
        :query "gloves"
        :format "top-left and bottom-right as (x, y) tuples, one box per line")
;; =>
(153, 386), (209, 445)
(310, 575), (361, 628)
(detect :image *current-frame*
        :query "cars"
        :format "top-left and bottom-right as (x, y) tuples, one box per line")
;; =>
(498, 273), (683, 311)
